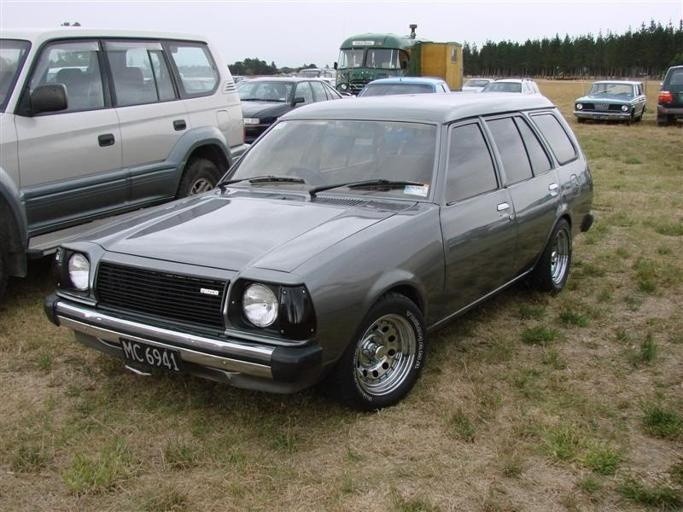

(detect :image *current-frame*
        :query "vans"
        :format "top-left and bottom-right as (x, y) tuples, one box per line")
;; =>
(296, 68), (336, 87)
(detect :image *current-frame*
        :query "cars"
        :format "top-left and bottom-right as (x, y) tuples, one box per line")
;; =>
(574, 79), (647, 125)
(350, 76), (450, 150)
(235, 79), (345, 143)
(462, 78), (494, 91)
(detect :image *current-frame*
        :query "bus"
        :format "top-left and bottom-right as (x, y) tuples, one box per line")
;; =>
(332, 23), (423, 93)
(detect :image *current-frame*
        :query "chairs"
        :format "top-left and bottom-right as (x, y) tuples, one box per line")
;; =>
(116, 67), (143, 89)
(56, 69), (82, 85)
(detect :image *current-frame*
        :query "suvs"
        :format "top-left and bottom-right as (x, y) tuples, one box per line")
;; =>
(482, 78), (541, 94)
(657, 65), (682, 127)
(0, 26), (252, 279)
(44, 92), (596, 413)
